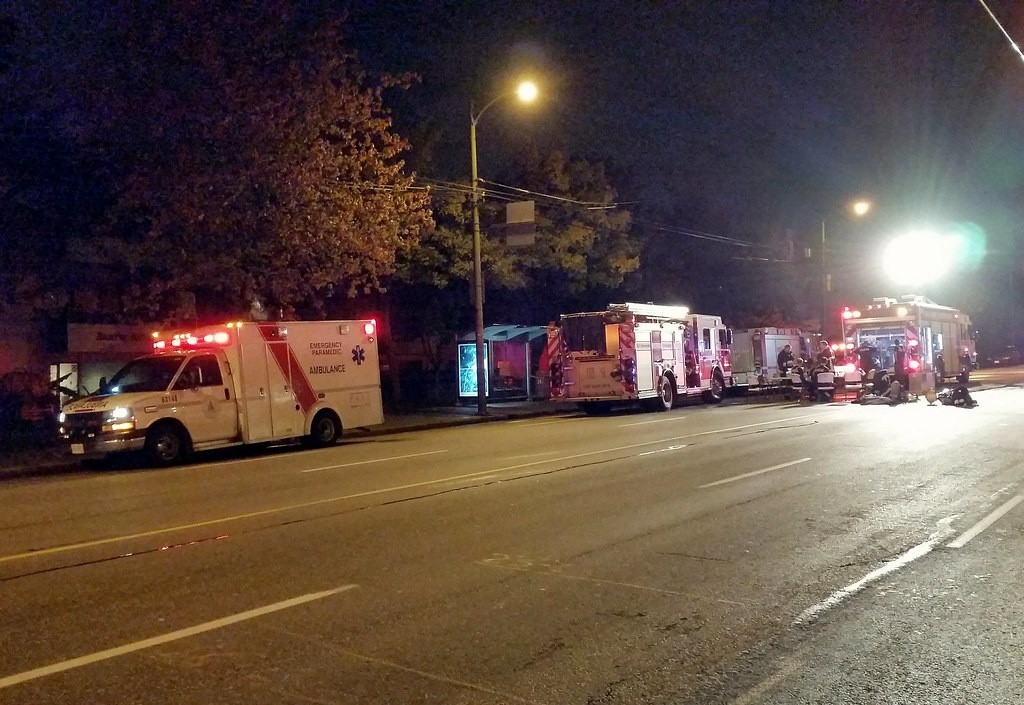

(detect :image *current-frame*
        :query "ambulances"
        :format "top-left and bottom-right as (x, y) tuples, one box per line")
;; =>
(57, 316), (385, 468)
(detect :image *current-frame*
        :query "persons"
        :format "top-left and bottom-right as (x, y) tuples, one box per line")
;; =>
(791, 358), (813, 396)
(783, 361), (793, 377)
(778, 344), (794, 377)
(893, 339), (906, 384)
(820, 341), (835, 374)
(798, 346), (812, 370)
(810, 357), (838, 403)
(853, 340), (877, 374)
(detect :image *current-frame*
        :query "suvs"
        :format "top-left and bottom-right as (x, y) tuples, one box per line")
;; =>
(987, 343), (1021, 367)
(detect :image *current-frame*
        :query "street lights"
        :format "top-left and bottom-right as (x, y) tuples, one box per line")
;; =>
(819, 197), (876, 334)
(469, 82), (542, 417)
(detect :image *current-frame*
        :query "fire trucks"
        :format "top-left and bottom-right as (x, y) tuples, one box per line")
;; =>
(837, 296), (978, 385)
(552, 302), (736, 411)
(719, 325), (846, 394)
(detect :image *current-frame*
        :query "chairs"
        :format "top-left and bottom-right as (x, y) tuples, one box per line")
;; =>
(746, 368), (877, 403)
